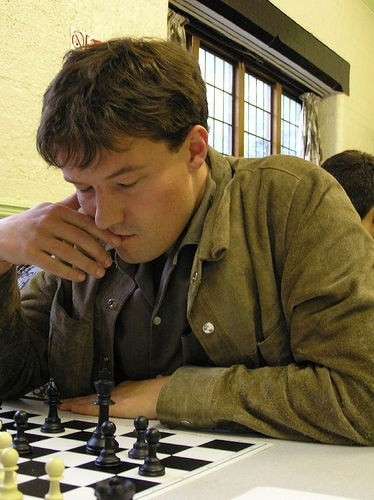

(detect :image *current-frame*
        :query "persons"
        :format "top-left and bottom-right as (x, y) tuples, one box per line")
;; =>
(0, 37), (374, 448)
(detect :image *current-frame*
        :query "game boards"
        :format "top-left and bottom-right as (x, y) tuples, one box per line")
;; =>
(0, 369), (255, 500)
